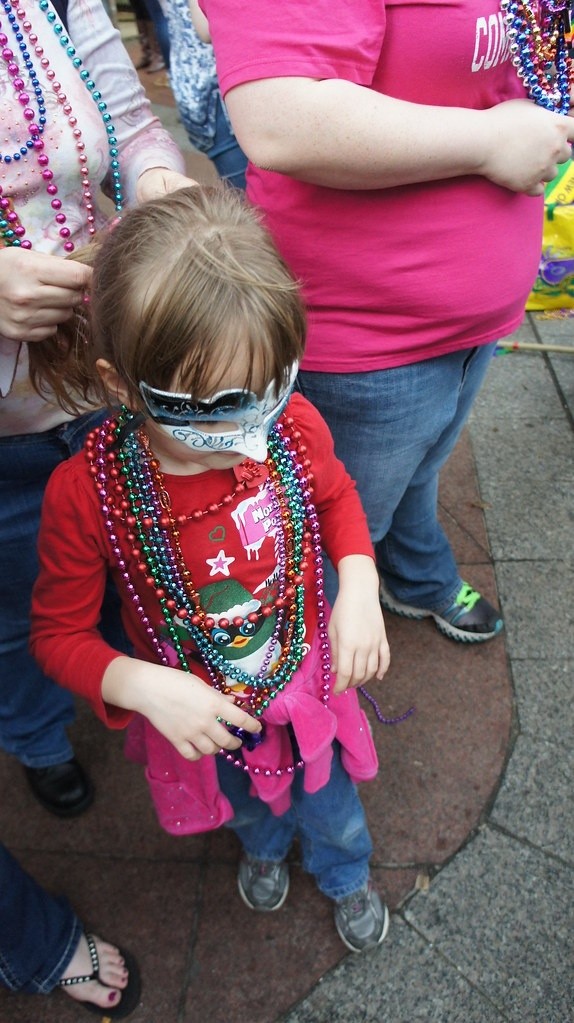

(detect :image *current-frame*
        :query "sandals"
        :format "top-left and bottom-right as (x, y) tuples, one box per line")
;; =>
(55, 927), (142, 1020)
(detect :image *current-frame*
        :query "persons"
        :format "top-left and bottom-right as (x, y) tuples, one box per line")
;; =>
(0, 844), (142, 1023)
(168, 0), (245, 193)
(104, 0), (172, 74)
(197, 1), (574, 641)
(0, 0), (197, 816)
(28, 185), (391, 955)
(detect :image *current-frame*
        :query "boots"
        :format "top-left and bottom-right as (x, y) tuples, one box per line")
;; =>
(148, 18), (171, 73)
(133, 19), (152, 70)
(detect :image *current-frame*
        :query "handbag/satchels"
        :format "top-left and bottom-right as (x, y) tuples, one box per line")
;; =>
(525, 158), (574, 313)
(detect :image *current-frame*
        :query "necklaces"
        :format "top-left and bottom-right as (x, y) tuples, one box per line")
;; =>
(499, 0), (574, 115)
(86, 406), (333, 777)
(0, 0), (124, 308)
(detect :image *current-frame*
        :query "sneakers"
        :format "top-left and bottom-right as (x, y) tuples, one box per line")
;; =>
(237, 854), (290, 911)
(23, 755), (95, 820)
(380, 575), (503, 643)
(333, 876), (390, 954)
